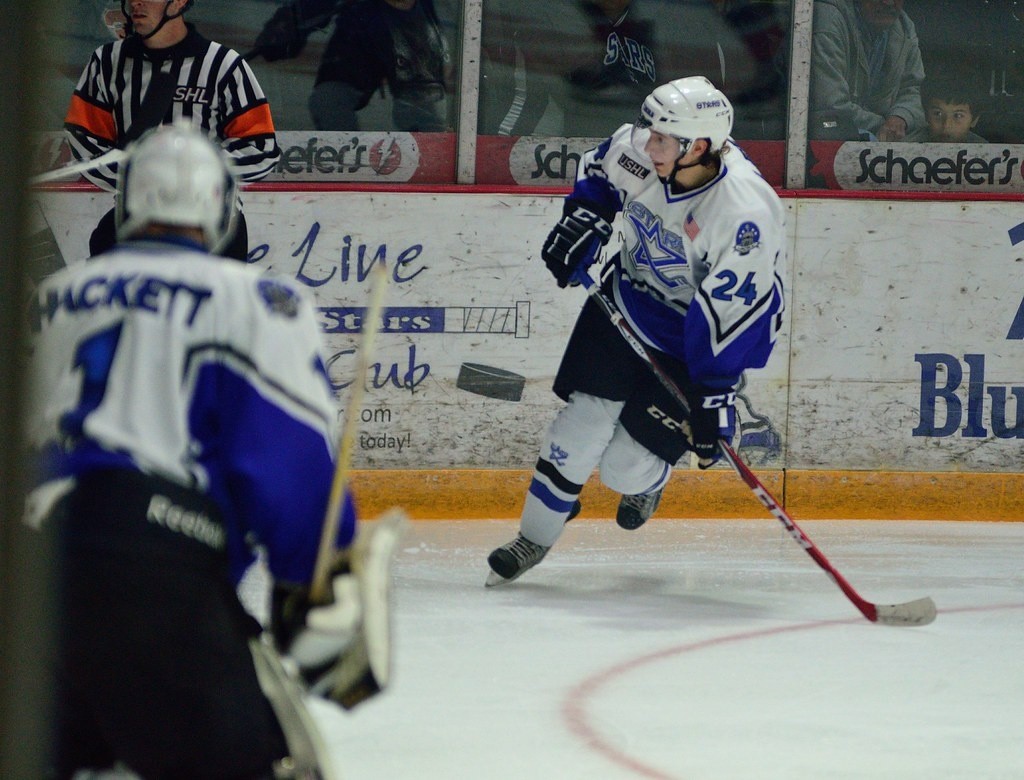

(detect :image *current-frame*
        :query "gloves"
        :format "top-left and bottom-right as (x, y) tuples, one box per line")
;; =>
(678, 386), (737, 470)
(541, 196), (616, 290)
(268, 553), (360, 670)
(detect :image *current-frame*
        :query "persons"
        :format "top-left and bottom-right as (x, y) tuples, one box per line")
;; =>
(485, 76), (786, 588)
(478, 0), (791, 139)
(807, 0), (928, 145)
(63, 0), (280, 262)
(22, 118), (362, 780)
(900, 68), (992, 145)
(257, 0), (452, 132)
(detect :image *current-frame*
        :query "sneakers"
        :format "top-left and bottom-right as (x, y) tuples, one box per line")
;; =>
(484, 532), (551, 588)
(616, 485), (664, 531)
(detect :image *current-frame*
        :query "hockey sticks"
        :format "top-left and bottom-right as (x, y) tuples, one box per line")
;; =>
(304, 272), (389, 596)
(582, 271), (938, 629)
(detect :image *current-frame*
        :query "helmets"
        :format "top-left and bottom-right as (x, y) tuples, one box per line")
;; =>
(638, 75), (734, 167)
(113, 118), (237, 252)
